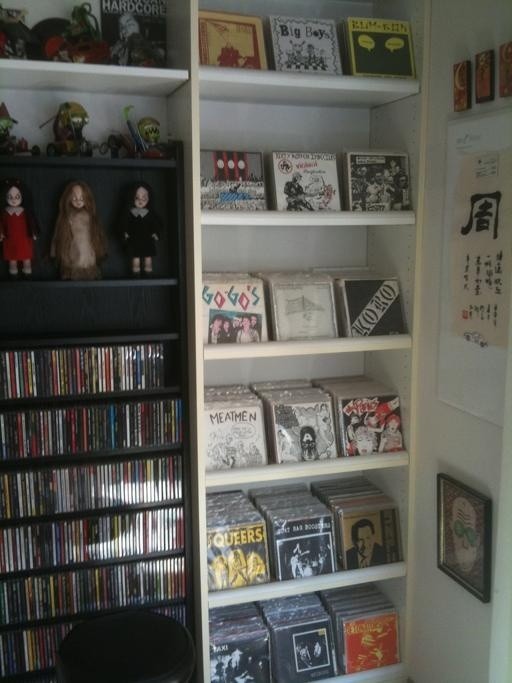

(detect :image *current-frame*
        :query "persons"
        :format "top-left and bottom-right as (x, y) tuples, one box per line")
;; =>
(119, 177), (161, 277)
(0, 174), (42, 279)
(49, 178), (111, 280)
(109, 10), (166, 67)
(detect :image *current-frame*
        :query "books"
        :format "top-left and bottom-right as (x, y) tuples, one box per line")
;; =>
(201, 375), (405, 473)
(201, 269), (410, 345)
(197, 6), (417, 79)
(100, 0), (170, 68)
(0, 342), (188, 683)
(200, 473), (406, 585)
(201, 147), (413, 213)
(202, 585), (418, 683)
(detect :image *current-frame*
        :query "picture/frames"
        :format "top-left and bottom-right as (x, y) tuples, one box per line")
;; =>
(437, 472), (492, 604)
